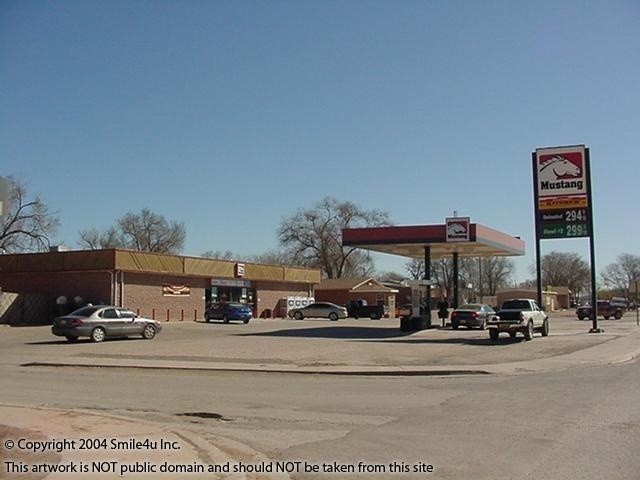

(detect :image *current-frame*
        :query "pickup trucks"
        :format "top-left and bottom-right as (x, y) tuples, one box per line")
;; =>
(340, 300), (386, 320)
(487, 298), (549, 341)
(576, 300), (627, 320)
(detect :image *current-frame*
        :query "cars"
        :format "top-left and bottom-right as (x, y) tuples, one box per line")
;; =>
(395, 304), (425, 318)
(289, 302), (348, 321)
(51, 305), (163, 343)
(204, 301), (252, 324)
(451, 304), (499, 330)
